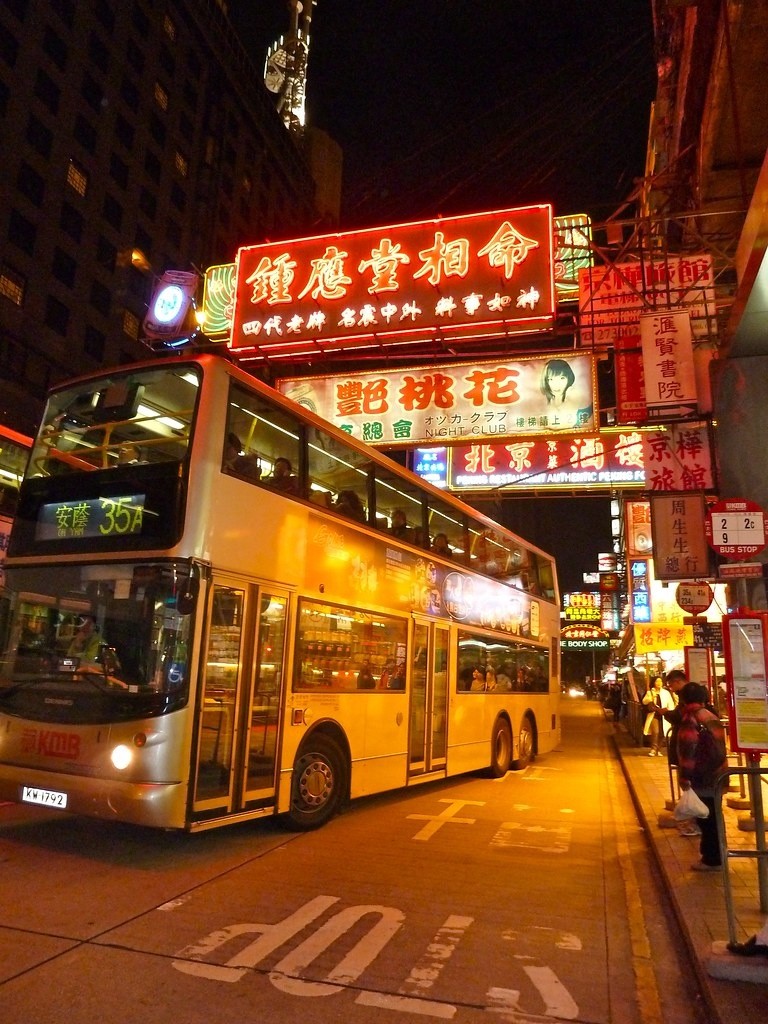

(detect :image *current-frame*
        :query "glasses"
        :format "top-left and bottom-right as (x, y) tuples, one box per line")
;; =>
(224, 445), (233, 451)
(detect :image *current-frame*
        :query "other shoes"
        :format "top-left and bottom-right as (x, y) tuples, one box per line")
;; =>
(613, 716), (620, 722)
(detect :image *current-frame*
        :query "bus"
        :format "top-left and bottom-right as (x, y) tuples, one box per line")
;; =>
(0, 352), (561, 833)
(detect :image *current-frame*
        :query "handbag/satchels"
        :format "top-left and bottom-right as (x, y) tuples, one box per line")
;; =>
(672, 787), (710, 824)
(694, 723), (726, 779)
(641, 690), (656, 714)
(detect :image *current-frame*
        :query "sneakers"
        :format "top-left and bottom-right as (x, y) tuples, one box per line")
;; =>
(648, 749), (664, 757)
(679, 823), (702, 837)
(690, 859), (723, 872)
(726, 934), (768, 957)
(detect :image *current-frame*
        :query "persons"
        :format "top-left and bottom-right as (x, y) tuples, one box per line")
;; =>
(658, 671), (730, 870)
(225, 432), (258, 478)
(642, 676), (676, 756)
(111, 441), (149, 468)
(38, 615), (125, 682)
(265, 456), (298, 494)
(461, 660), (549, 692)
(541, 359), (575, 415)
(611, 678), (630, 723)
(414, 526), (423, 546)
(357, 659), (405, 689)
(323, 490), (367, 523)
(432, 533), (452, 559)
(391, 511), (414, 542)
